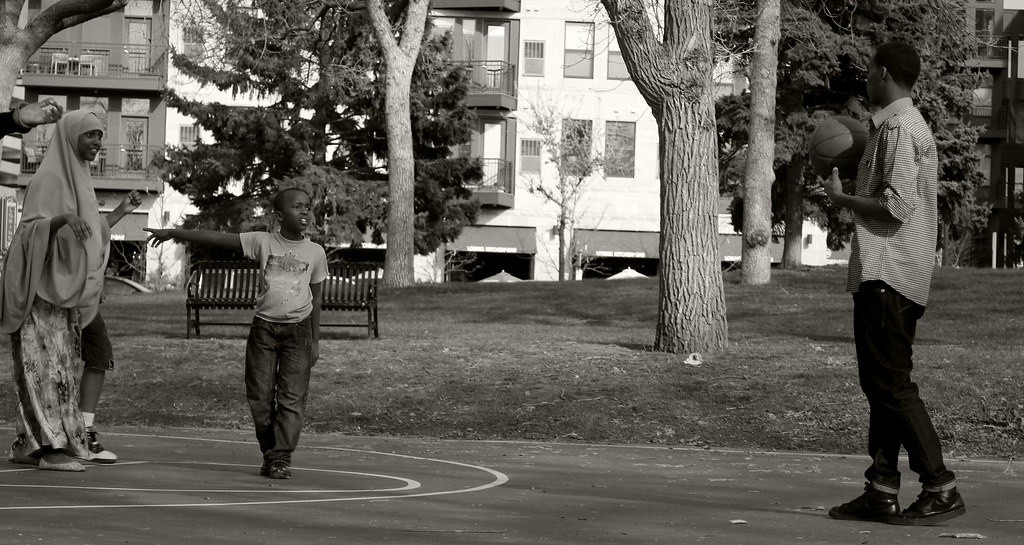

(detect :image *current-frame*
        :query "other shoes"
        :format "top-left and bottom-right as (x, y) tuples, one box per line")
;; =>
(74, 428), (118, 462)
(270, 461), (291, 478)
(828, 481), (901, 523)
(259, 462), (271, 477)
(39, 454), (86, 472)
(887, 485), (967, 526)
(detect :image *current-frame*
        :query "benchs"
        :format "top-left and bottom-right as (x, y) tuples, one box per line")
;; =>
(185, 259), (379, 340)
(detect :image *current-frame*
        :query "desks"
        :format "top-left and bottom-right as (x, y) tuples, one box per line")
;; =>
(68, 57), (78, 74)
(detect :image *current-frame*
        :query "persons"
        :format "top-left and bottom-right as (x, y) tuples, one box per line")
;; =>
(143, 188), (328, 479)
(0, 98), (142, 472)
(826, 41), (967, 524)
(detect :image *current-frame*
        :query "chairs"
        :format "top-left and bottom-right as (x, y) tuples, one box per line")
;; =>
(24, 147), (43, 170)
(78, 55), (97, 76)
(50, 52), (69, 75)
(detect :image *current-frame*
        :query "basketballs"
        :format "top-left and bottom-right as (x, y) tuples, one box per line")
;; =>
(807, 114), (870, 178)
